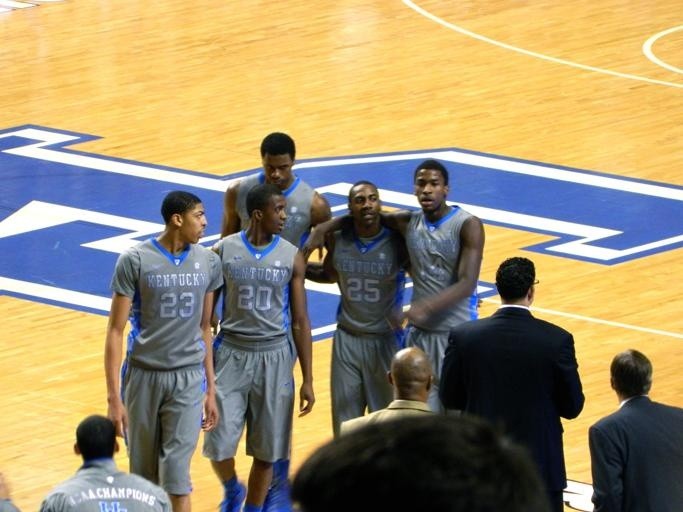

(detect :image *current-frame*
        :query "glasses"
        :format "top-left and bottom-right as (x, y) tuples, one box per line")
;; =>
(529, 279), (540, 286)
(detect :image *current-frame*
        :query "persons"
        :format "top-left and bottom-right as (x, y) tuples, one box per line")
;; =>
(201, 184), (316, 512)
(39, 415), (173, 511)
(438, 256), (585, 511)
(212, 133), (333, 332)
(590, 348), (683, 512)
(302, 160), (485, 413)
(306, 180), (483, 438)
(290, 348), (552, 512)
(100, 192), (224, 511)
(0, 472), (19, 512)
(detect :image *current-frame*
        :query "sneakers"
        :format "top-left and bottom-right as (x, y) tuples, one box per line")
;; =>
(221, 460), (292, 512)
